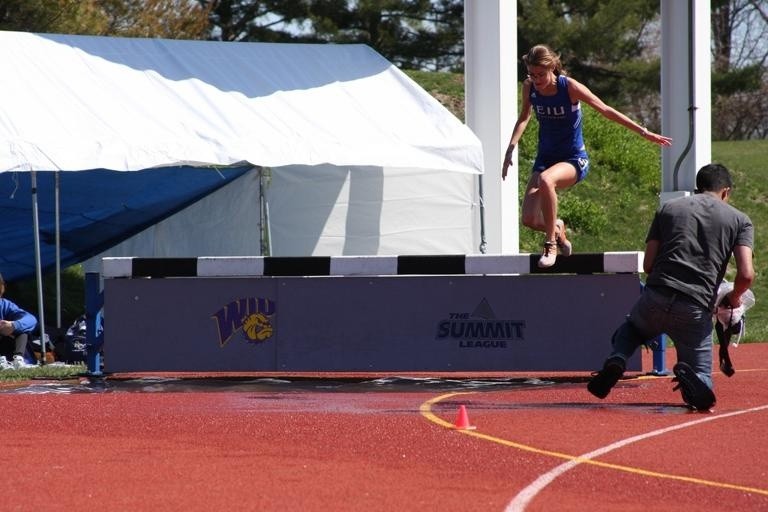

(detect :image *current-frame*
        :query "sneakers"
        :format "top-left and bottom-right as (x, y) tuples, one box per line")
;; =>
(587, 353), (626, 399)
(11, 355), (40, 370)
(0, 355), (16, 371)
(537, 239), (557, 269)
(673, 361), (716, 410)
(555, 218), (572, 258)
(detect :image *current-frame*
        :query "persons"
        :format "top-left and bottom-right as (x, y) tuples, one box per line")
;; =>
(500, 44), (674, 269)
(0, 274), (39, 370)
(588, 164), (755, 411)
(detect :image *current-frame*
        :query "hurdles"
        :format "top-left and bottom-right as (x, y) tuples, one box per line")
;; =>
(70, 251), (671, 388)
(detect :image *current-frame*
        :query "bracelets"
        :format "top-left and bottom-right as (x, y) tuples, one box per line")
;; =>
(642, 125), (649, 137)
